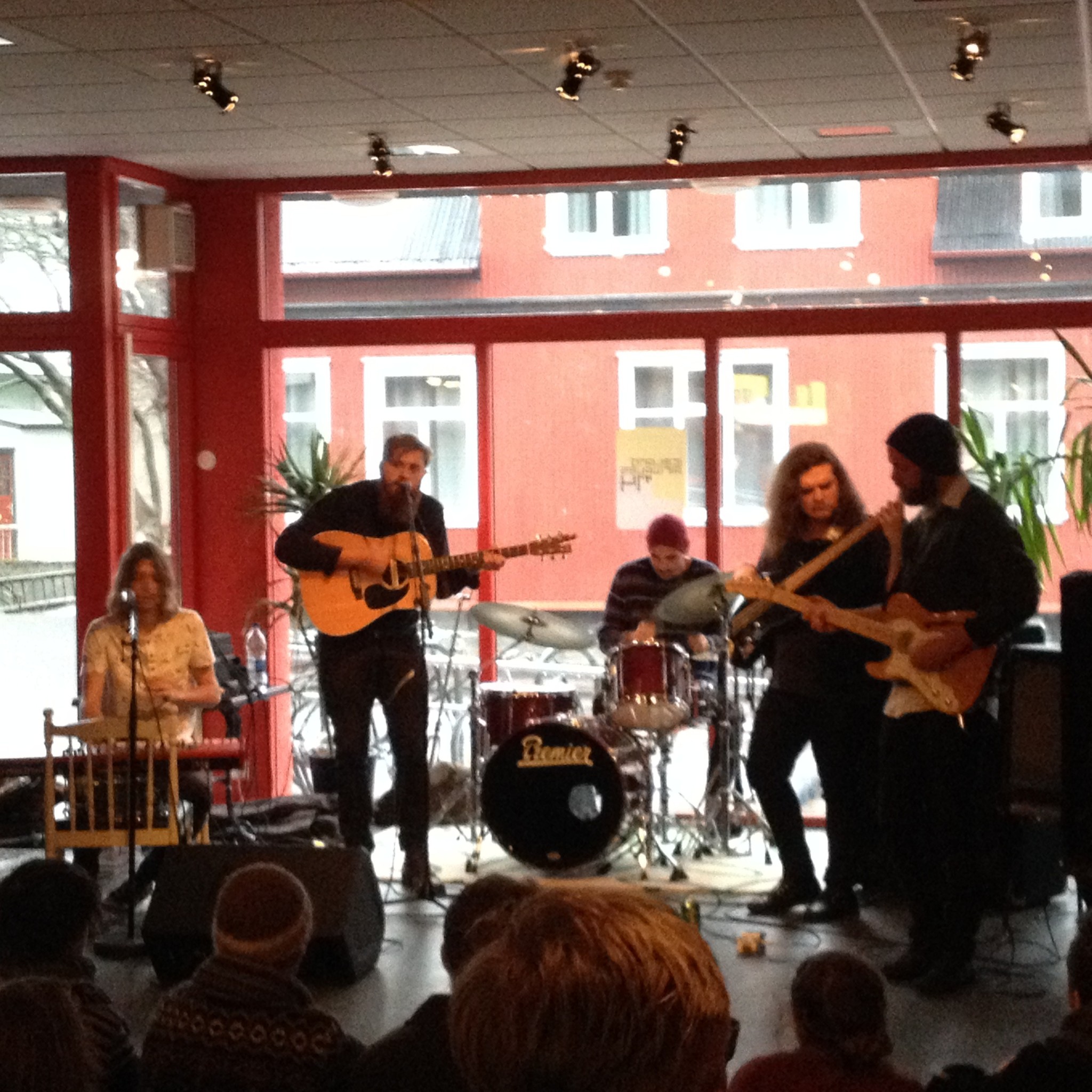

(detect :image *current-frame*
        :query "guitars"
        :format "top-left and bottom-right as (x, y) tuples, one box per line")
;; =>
(298, 529), (578, 636)
(727, 499), (905, 666)
(723, 577), (995, 712)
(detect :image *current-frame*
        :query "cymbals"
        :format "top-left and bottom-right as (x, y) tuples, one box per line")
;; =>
(469, 603), (590, 651)
(655, 570), (739, 626)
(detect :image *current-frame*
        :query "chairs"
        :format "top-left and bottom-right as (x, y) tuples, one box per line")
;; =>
(43, 706), (208, 853)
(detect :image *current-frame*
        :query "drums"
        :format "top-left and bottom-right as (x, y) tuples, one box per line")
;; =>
(603, 640), (693, 732)
(480, 716), (652, 870)
(478, 679), (577, 744)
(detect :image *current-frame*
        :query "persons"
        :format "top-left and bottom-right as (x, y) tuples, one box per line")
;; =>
(0, 858), (141, 1092)
(801, 414), (1042, 995)
(729, 948), (924, 1092)
(132, 863), (368, 1092)
(730, 439), (905, 927)
(73, 541), (222, 941)
(364, 873), (550, 1092)
(926, 917), (1092, 1092)
(591, 514), (744, 837)
(445, 875), (739, 1091)
(274, 432), (508, 897)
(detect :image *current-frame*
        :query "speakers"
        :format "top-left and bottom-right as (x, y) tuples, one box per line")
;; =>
(1000, 639), (1064, 831)
(141, 843), (385, 992)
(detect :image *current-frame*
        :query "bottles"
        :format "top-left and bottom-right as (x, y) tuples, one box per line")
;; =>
(244, 623), (268, 693)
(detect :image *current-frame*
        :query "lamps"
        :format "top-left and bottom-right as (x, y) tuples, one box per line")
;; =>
(948, 27), (993, 83)
(366, 130), (398, 178)
(663, 117), (701, 163)
(185, 56), (242, 117)
(985, 100), (1028, 145)
(552, 37), (607, 102)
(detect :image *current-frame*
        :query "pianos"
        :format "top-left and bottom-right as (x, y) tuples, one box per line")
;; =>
(0, 737), (246, 777)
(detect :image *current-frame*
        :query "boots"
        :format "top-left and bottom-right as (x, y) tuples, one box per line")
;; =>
(401, 837), (446, 896)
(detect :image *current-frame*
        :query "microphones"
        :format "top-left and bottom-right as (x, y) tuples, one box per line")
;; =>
(127, 606), (141, 639)
(398, 479), (414, 504)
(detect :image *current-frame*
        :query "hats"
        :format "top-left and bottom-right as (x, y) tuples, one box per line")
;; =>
(210, 860), (314, 968)
(645, 513), (690, 556)
(885, 413), (959, 477)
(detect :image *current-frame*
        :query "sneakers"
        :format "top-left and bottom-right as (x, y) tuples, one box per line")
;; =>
(882, 928), (982, 995)
(746, 874), (862, 923)
